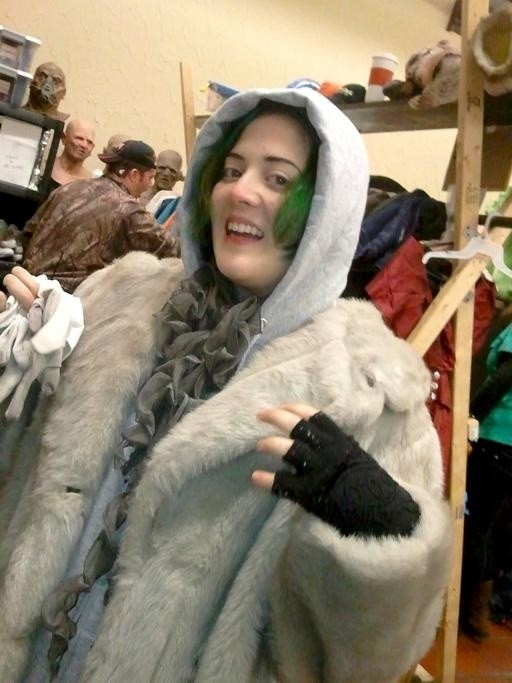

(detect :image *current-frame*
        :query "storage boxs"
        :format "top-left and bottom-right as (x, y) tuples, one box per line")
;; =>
(0, 24), (42, 70)
(0, 63), (34, 105)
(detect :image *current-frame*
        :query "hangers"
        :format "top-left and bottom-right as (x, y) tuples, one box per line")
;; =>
(420, 208), (511, 281)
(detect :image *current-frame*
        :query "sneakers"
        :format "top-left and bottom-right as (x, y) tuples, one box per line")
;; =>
(458, 597), (512, 640)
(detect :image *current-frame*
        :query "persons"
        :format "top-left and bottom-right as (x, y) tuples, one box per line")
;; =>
(25, 59), (71, 126)
(19, 138), (181, 295)
(50, 116), (97, 192)
(150, 149), (186, 200)
(457, 308), (511, 644)
(0, 86), (458, 683)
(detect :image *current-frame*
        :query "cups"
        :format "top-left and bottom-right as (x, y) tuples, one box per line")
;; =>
(363, 53), (400, 104)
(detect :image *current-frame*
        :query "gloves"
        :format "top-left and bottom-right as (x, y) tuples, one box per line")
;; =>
(270, 410), (420, 539)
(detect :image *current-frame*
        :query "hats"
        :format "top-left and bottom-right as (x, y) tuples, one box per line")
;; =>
(99, 138), (159, 168)
